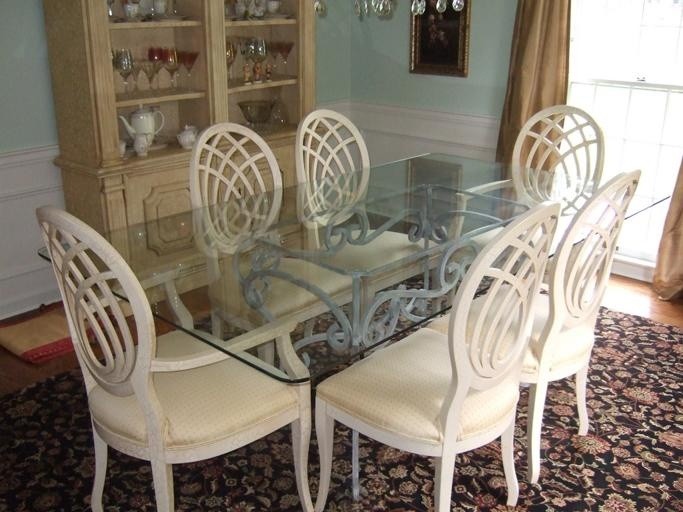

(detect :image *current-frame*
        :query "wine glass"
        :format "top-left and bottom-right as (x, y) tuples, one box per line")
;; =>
(106, 0), (294, 100)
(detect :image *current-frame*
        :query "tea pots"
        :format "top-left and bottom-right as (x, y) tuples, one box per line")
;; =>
(117, 104), (201, 161)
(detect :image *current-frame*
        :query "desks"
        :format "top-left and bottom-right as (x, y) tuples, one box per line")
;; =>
(37, 152), (672, 496)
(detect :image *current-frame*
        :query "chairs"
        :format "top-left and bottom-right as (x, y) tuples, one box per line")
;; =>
(452, 103), (612, 302)
(427, 167), (648, 486)
(37, 203), (322, 512)
(311, 198), (564, 511)
(295, 104), (444, 341)
(189, 122), (362, 368)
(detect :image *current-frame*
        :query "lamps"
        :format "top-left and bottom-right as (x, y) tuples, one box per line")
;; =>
(313, 0), (465, 17)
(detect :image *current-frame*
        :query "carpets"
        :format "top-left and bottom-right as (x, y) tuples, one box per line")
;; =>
(0, 300), (114, 366)
(0, 262), (683, 512)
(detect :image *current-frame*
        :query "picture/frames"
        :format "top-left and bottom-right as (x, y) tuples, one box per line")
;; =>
(409, 0), (472, 77)
(404, 157), (464, 235)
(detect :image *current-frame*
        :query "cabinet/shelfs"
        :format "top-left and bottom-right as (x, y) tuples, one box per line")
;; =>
(43, 1), (331, 317)
